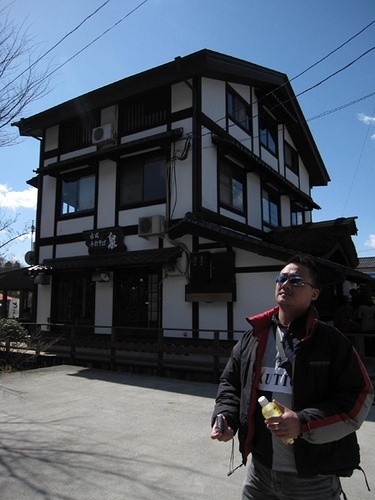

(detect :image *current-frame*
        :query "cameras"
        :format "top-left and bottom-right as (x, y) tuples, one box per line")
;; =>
(215, 415), (226, 435)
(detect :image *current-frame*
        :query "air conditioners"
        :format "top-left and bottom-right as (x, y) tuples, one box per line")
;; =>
(138, 215), (166, 238)
(92, 124), (116, 146)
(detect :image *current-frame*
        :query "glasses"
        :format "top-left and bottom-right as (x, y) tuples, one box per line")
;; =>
(274, 275), (315, 288)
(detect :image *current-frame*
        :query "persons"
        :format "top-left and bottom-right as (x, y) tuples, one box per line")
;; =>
(211, 256), (374, 500)
(333, 289), (375, 357)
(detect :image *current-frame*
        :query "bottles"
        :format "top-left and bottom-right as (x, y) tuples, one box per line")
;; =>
(258, 396), (297, 445)
